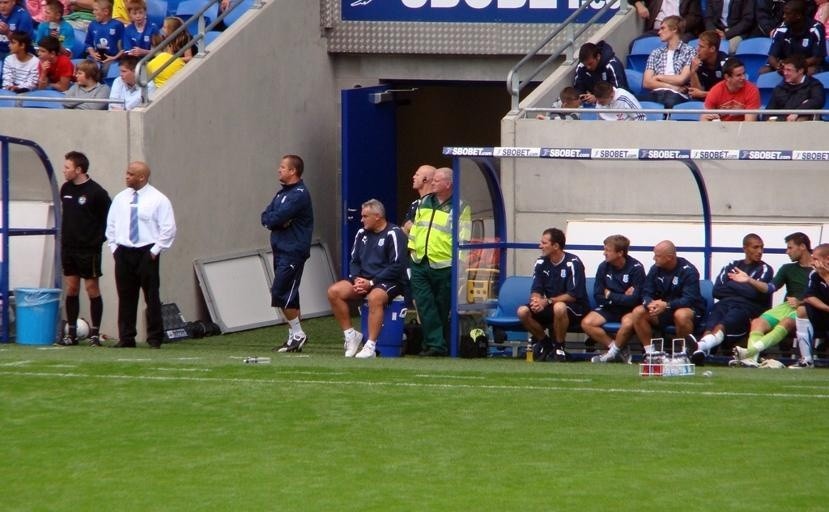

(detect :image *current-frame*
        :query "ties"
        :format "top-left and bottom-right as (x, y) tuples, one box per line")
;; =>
(129, 191), (139, 244)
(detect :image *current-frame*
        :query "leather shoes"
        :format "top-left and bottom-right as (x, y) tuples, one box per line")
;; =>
(114, 336), (136, 347)
(151, 342), (161, 349)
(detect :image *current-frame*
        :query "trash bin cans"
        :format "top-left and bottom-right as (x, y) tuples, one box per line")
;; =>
(13, 287), (63, 347)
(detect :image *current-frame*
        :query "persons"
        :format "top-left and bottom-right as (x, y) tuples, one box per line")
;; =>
(518, 227), (829, 367)
(325, 163), (474, 359)
(257, 152), (317, 356)
(1, 0), (236, 112)
(103, 161), (177, 351)
(535, 0), (829, 122)
(52, 149), (114, 350)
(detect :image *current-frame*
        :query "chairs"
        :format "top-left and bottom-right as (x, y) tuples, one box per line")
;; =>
(0, 1), (253, 112)
(480, 273), (532, 360)
(549, 276), (597, 361)
(664, 278), (713, 367)
(721, 326), (828, 367)
(598, 321), (633, 332)
(572, 32), (828, 121)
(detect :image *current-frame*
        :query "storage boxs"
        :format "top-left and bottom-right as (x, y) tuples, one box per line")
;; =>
(359, 292), (408, 359)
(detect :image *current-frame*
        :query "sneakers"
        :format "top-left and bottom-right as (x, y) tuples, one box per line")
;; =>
(271, 330), (377, 358)
(418, 348), (447, 356)
(53, 334), (81, 346)
(88, 336), (102, 348)
(530, 340), (816, 369)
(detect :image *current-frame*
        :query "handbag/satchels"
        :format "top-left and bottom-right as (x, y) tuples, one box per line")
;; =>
(403, 318), (423, 354)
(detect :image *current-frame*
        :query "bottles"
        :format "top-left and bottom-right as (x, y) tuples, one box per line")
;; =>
(242, 358), (270, 365)
(526, 337), (533, 363)
(643, 352), (693, 377)
